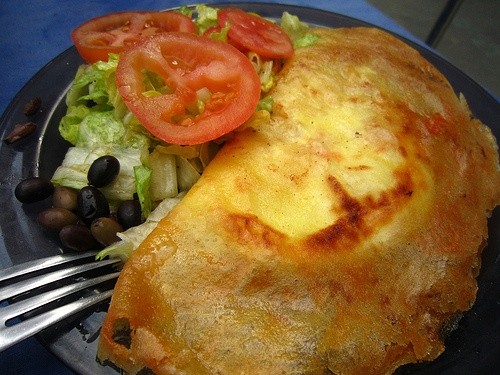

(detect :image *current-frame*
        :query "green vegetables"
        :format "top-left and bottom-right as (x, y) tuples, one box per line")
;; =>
(52, 37), (278, 261)
(172, 2), (322, 48)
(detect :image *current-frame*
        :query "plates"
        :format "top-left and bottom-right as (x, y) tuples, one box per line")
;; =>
(1, 3), (499, 375)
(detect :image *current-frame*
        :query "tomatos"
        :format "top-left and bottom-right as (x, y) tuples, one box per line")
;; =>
(71, 9), (296, 143)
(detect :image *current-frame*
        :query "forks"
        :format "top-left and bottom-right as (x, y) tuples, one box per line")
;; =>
(0, 253), (120, 350)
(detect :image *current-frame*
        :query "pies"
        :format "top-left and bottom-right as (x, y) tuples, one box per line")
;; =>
(99, 28), (500, 374)
